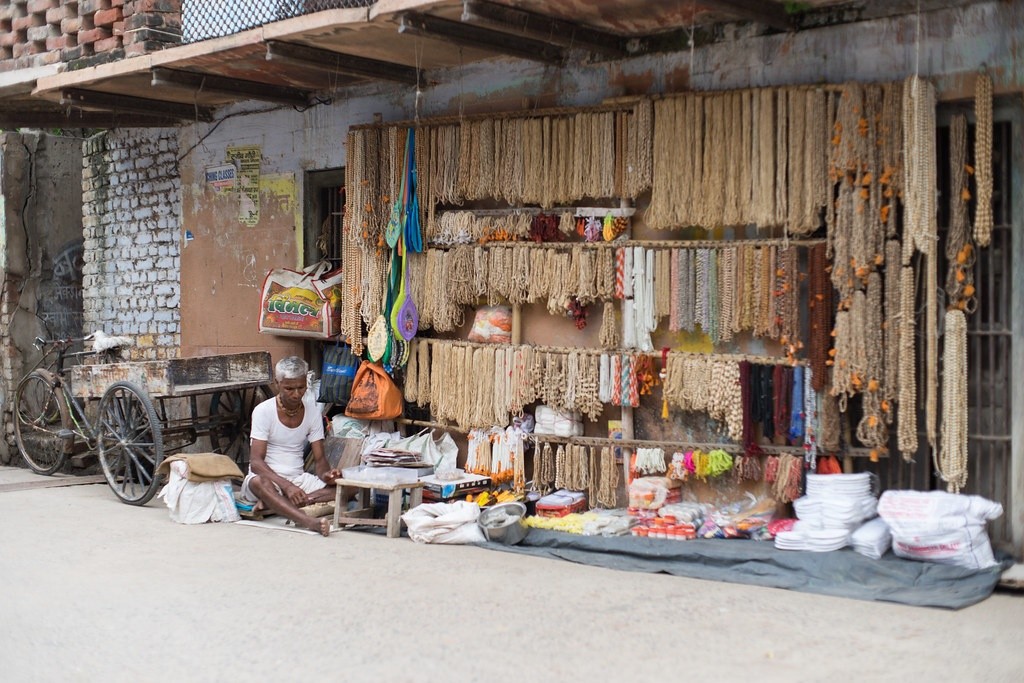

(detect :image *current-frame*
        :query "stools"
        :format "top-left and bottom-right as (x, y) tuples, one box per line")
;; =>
(333, 478), (425, 537)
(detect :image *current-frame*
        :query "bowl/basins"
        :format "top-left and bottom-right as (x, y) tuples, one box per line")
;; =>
(477, 502), (529, 545)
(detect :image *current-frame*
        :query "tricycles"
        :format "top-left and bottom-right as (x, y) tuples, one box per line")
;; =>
(12, 328), (275, 507)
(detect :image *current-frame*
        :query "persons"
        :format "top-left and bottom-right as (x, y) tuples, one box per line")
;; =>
(240, 356), (359, 537)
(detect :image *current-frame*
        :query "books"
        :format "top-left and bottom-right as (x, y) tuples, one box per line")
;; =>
(418, 472), (492, 503)
